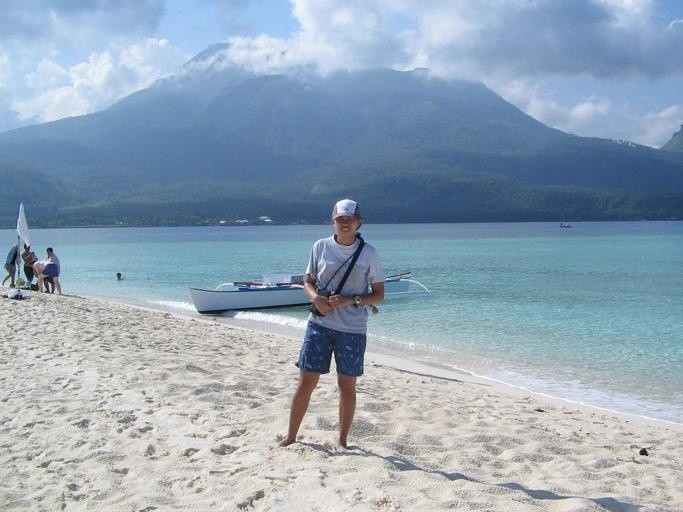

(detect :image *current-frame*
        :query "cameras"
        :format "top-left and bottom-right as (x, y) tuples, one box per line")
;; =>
(309, 290), (330, 318)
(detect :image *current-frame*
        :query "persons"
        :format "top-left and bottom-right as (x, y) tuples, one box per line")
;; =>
(116, 273), (123, 282)
(2, 246), (21, 288)
(43, 247), (60, 294)
(21, 244), (38, 290)
(30, 259), (62, 295)
(268, 198), (385, 452)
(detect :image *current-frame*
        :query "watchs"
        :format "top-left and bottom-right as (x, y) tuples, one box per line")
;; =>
(354, 295), (362, 306)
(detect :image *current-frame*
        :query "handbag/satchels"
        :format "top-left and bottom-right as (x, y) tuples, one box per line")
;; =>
(309, 289), (334, 317)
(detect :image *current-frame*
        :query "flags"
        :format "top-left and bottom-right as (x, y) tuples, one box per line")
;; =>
(16, 202), (31, 247)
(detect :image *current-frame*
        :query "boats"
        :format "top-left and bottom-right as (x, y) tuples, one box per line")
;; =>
(190, 272), (409, 314)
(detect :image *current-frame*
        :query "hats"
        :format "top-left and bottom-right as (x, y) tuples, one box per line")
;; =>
(331, 198), (361, 223)
